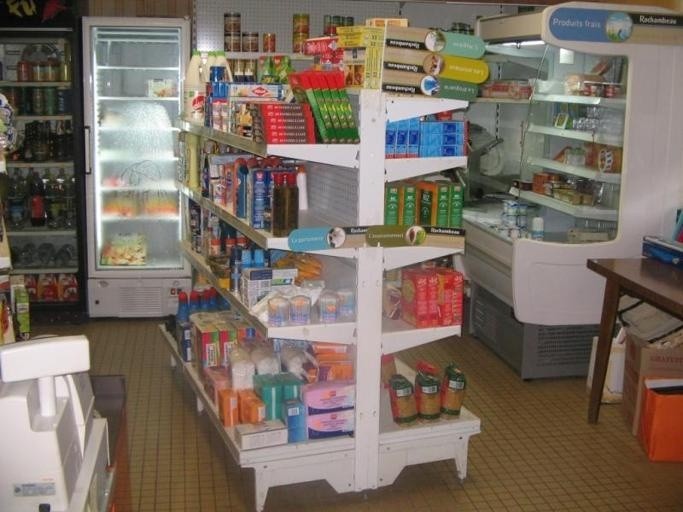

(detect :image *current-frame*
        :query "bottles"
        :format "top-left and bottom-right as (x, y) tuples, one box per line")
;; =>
(272, 171), (299, 236)
(5, 122), (76, 268)
(176, 288), (217, 353)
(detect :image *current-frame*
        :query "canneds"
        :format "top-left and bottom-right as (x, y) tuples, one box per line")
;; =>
(223, 12), (355, 52)
(17, 43), (72, 81)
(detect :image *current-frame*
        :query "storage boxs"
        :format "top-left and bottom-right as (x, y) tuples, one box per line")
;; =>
(586, 332), (683, 462)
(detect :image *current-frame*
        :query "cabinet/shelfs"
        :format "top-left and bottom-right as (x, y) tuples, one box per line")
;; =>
(461, 91), (625, 381)
(158, 77), (483, 512)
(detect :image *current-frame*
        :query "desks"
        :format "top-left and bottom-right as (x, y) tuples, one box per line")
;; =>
(585, 256), (683, 423)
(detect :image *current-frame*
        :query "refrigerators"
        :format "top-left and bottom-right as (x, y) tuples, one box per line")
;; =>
(0, 1), (84, 324)
(83, 17), (191, 321)
